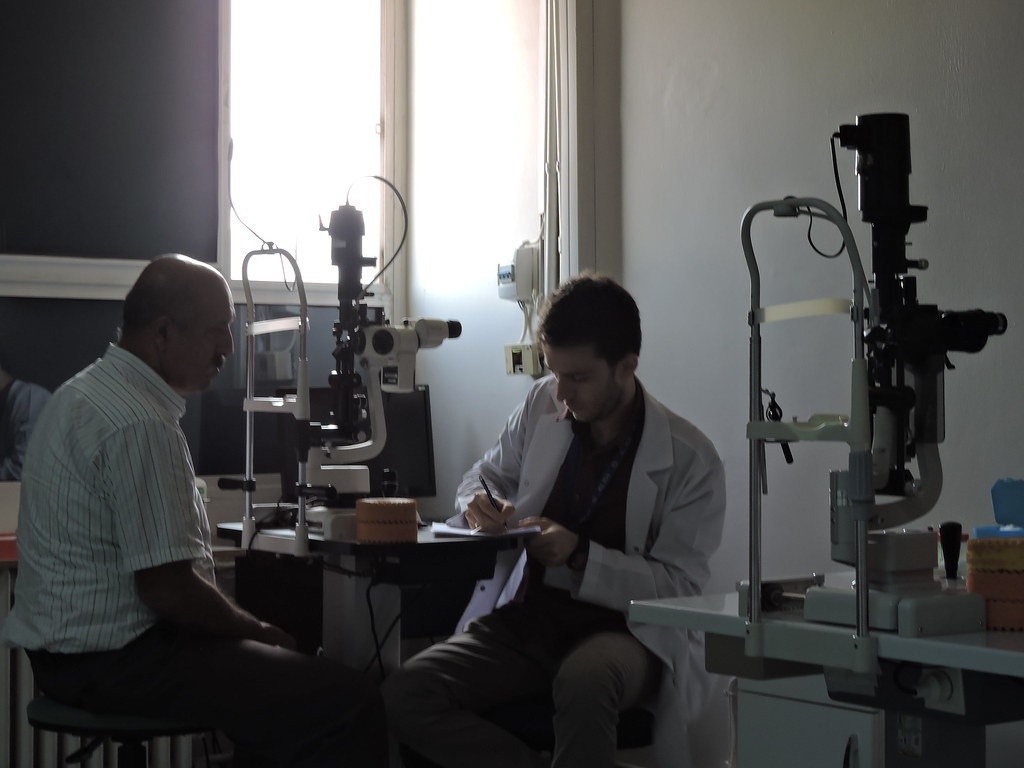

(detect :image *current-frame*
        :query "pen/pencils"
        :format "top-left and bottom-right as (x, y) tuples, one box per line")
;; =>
(476, 474), (507, 529)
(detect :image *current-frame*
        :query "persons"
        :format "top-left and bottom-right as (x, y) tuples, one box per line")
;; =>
(0, 371), (53, 482)
(377, 269), (730, 768)
(1, 252), (390, 768)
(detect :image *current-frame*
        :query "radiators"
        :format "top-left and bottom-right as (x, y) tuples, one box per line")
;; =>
(0, 530), (193, 768)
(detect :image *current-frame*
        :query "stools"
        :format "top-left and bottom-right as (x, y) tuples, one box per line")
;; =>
(26, 695), (218, 768)
